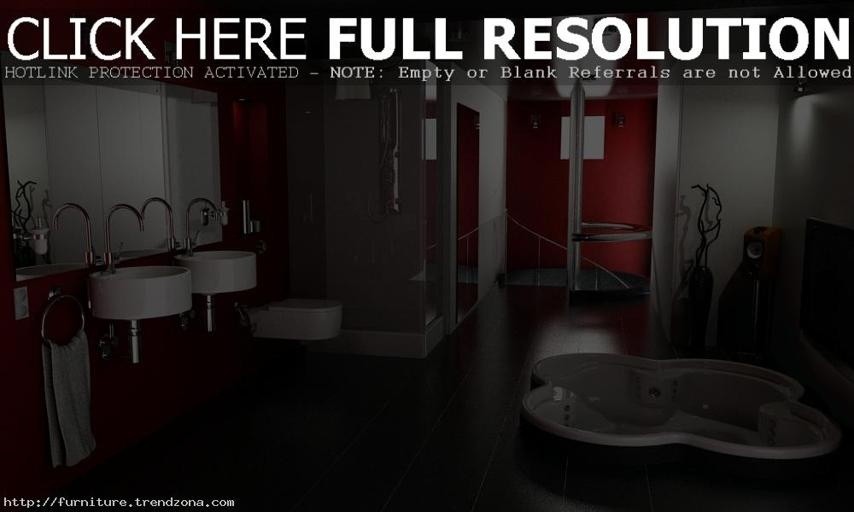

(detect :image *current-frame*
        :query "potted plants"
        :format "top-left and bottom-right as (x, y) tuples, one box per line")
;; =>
(15, 175), (38, 266)
(685, 175), (723, 346)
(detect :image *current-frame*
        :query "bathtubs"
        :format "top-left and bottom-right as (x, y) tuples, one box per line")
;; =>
(520, 350), (842, 463)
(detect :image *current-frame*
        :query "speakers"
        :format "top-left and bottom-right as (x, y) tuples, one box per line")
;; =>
(742, 226), (780, 267)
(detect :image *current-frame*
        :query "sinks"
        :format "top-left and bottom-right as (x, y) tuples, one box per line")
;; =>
(84, 266), (192, 321)
(174, 248), (258, 294)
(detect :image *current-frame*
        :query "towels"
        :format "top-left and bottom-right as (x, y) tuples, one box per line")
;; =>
(40, 328), (98, 468)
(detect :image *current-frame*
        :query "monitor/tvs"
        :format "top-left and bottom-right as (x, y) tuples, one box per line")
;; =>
(799, 216), (854, 387)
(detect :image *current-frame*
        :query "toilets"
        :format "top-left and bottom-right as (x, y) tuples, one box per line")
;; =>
(247, 296), (345, 343)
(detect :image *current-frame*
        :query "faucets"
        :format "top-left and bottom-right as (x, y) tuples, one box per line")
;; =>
(102, 201), (146, 273)
(140, 195), (176, 251)
(182, 195), (221, 257)
(51, 200), (95, 266)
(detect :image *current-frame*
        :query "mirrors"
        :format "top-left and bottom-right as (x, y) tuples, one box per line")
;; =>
(422, 59), (445, 324)
(2, 51), (224, 281)
